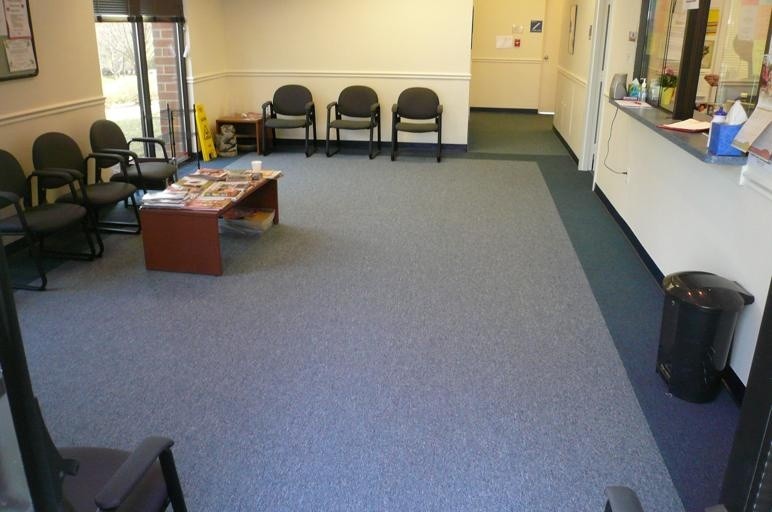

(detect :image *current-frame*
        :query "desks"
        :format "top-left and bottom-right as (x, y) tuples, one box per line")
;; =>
(139, 167), (283, 276)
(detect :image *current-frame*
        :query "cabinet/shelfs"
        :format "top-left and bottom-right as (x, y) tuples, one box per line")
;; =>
(216, 112), (273, 155)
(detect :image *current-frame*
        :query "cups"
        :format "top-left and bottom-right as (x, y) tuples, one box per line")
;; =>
(249, 160), (262, 173)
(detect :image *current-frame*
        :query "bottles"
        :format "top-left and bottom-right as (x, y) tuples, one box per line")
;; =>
(707, 105), (728, 147)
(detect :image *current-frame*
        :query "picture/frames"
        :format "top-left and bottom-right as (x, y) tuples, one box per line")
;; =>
(700, 40), (714, 69)
(529, 19), (542, 33)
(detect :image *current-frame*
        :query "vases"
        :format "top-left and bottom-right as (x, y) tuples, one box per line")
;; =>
(660, 87), (675, 108)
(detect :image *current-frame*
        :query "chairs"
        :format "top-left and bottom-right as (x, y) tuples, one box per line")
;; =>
(0, 120), (177, 291)
(33, 396), (189, 511)
(262, 85), (444, 163)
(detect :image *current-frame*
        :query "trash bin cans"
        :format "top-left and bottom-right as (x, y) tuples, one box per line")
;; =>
(655, 271), (754, 403)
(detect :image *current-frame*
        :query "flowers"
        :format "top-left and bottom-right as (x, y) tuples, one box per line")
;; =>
(655, 67), (679, 88)
